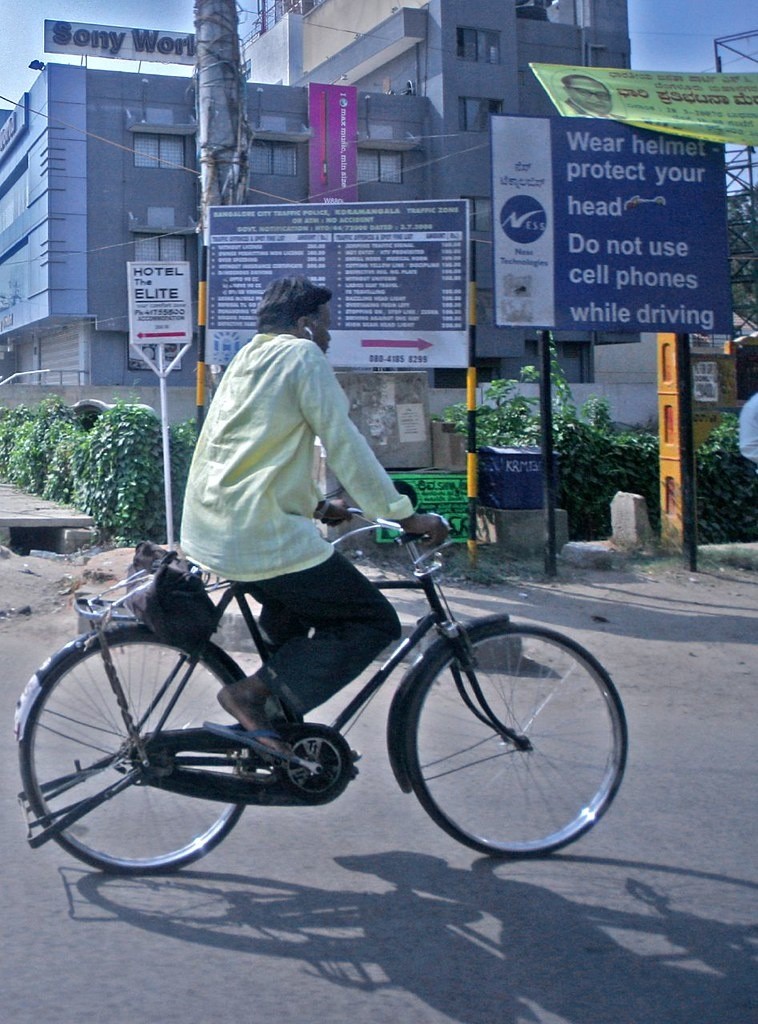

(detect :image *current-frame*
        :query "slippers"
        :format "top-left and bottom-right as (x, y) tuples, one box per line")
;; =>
(350, 747), (362, 762)
(203, 721), (301, 763)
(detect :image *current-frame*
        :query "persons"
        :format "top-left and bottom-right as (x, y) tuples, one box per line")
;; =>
(738, 392), (758, 466)
(180, 277), (449, 758)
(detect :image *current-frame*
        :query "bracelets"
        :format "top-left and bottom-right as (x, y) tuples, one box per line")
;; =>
(314, 499), (331, 520)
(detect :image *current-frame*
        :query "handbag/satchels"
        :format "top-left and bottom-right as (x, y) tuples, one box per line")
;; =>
(125, 540), (217, 653)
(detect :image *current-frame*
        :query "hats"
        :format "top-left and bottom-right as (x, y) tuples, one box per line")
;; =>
(256, 273), (335, 317)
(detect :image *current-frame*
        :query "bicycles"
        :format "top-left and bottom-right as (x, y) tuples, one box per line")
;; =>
(13, 508), (629, 876)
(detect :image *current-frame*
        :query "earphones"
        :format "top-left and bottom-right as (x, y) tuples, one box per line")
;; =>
(303, 326), (313, 336)
